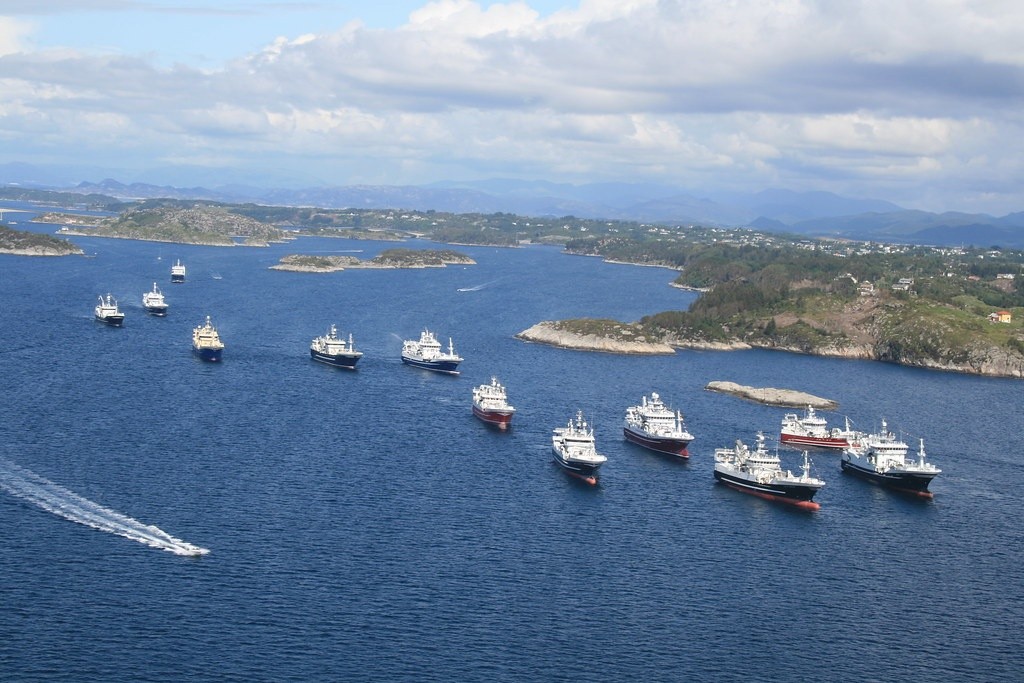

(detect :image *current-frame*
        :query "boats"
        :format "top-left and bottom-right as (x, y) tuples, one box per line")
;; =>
(780, 405), (866, 451)
(840, 420), (943, 500)
(171, 259), (186, 285)
(310, 323), (362, 369)
(621, 391), (694, 460)
(552, 408), (607, 485)
(94, 291), (124, 329)
(471, 375), (516, 431)
(400, 328), (465, 376)
(712, 430), (826, 514)
(192, 314), (226, 362)
(143, 281), (170, 318)
